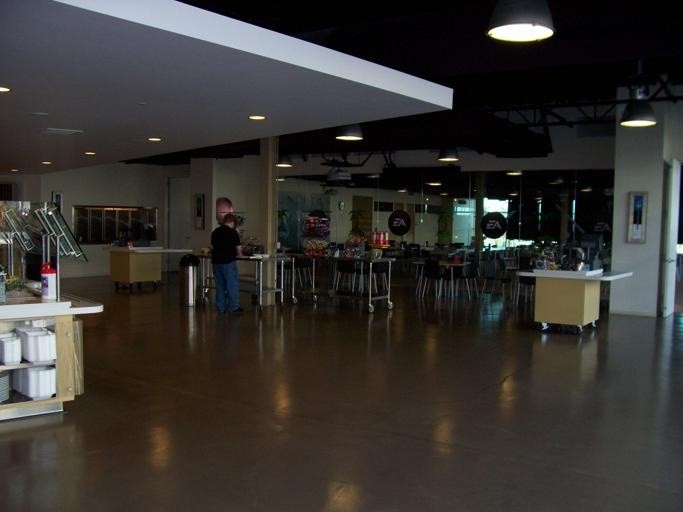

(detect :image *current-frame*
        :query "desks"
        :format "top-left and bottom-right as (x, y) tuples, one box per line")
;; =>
(99, 247), (291, 316)
(515, 267), (634, 335)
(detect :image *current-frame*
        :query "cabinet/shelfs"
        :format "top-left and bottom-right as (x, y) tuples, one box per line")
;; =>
(1, 314), (87, 422)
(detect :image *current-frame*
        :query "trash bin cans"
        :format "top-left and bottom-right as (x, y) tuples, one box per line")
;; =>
(179, 253), (200, 307)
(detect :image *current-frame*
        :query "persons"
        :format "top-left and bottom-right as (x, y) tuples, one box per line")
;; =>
(210, 215), (243, 314)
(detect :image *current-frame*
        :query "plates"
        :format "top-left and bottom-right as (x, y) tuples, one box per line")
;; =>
(0, 325), (56, 403)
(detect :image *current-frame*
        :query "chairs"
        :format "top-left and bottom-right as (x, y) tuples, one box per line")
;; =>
(276, 240), (531, 314)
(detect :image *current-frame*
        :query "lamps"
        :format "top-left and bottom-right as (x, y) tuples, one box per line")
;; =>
(620, 81), (657, 128)
(487, 1), (557, 45)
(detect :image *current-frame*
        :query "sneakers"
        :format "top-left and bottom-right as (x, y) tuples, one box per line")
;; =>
(220, 307), (243, 313)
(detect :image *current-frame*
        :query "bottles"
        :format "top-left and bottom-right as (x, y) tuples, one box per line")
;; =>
(39, 262), (56, 300)
